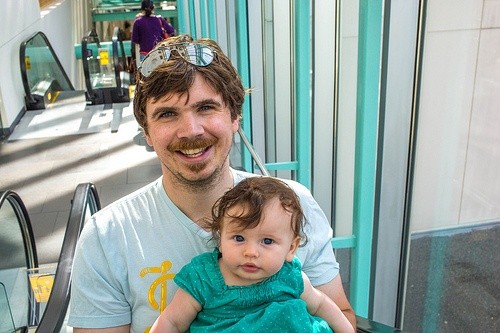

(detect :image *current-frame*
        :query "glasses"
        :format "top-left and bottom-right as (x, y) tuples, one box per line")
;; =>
(138, 42), (221, 77)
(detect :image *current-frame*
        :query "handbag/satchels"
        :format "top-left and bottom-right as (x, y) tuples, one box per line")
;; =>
(158, 17), (169, 39)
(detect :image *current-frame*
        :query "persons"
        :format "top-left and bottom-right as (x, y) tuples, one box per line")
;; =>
(148, 176), (356, 333)
(66, 34), (357, 333)
(120, 20), (132, 40)
(131, 0), (176, 62)
